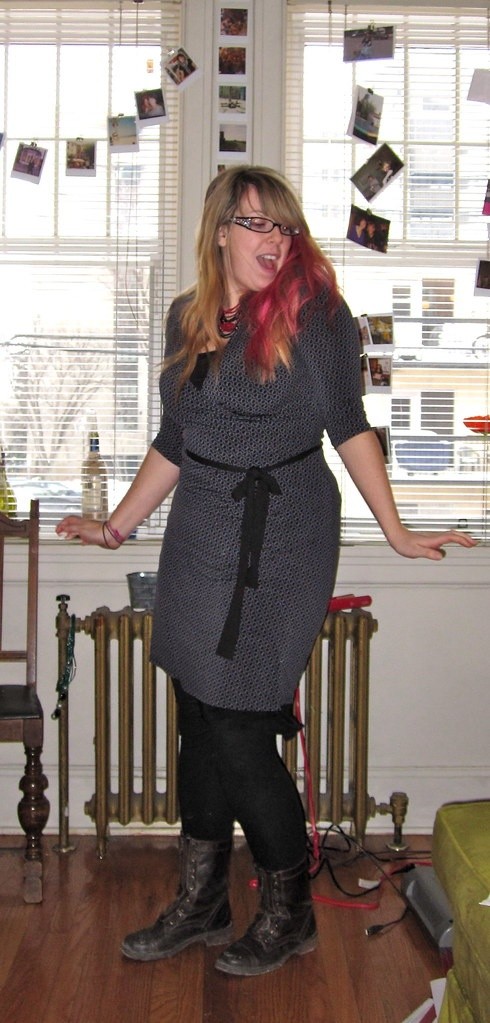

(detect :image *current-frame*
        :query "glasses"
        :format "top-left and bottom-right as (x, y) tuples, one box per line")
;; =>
(228, 217), (301, 236)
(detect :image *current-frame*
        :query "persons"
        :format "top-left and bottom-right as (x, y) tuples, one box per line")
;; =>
(350, 217), (384, 251)
(28, 156), (34, 174)
(55, 167), (477, 976)
(177, 54), (196, 76)
(141, 95), (163, 116)
(372, 364), (388, 386)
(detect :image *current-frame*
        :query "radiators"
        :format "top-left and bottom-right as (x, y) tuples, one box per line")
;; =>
(51, 594), (411, 852)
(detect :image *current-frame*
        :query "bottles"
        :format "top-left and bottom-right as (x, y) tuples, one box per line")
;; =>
(0, 453), (17, 517)
(80, 431), (107, 522)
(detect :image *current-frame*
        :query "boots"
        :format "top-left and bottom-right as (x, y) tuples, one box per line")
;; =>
(118, 832), (233, 961)
(215, 851), (319, 976)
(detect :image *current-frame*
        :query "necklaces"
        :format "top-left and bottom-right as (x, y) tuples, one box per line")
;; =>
(216, 305), (239, 338)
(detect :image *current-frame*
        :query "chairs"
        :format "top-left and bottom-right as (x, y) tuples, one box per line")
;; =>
(459, 447), (481, 473)
(0, 499), (54, 904)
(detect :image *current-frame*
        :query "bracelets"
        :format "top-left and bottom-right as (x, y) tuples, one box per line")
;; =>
(102, 522), (122, 549)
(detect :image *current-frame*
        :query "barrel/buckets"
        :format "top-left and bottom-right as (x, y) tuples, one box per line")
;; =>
(126, 572), (159, 612)
